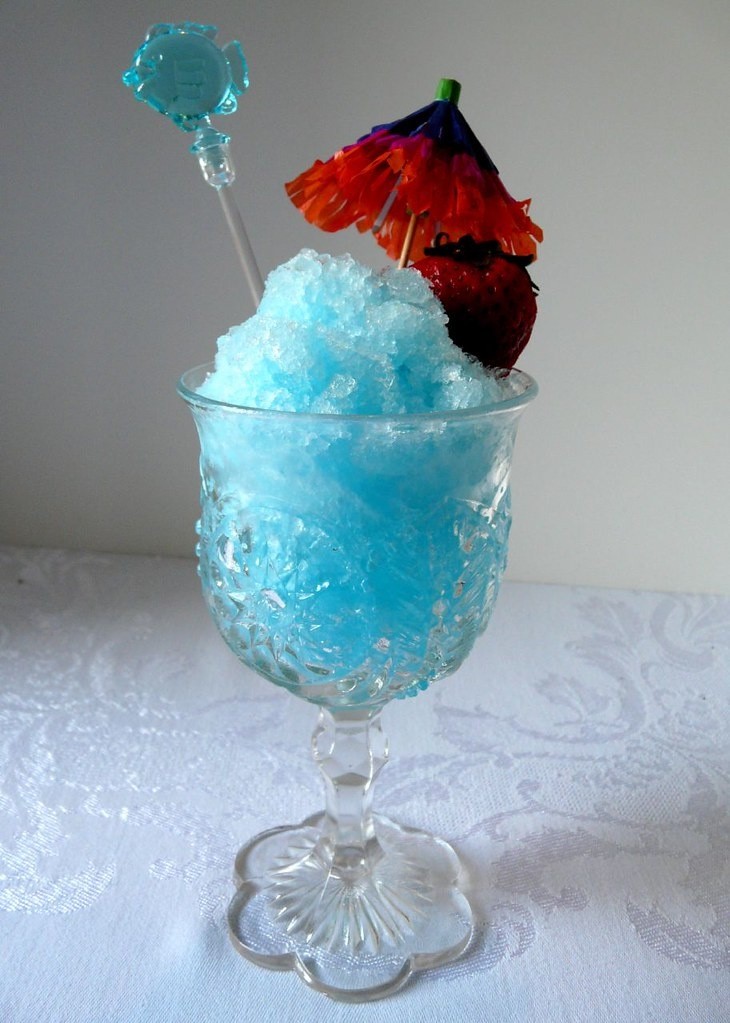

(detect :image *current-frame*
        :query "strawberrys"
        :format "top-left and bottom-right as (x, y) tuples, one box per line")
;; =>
(404, 234), (538, 379)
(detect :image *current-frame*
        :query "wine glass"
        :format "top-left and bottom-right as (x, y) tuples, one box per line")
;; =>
(176, 357), (541, 1005)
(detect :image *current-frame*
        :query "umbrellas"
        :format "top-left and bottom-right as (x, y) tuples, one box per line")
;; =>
(285, 77), (542, 269)
